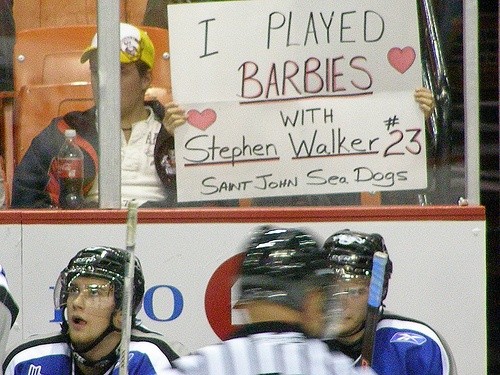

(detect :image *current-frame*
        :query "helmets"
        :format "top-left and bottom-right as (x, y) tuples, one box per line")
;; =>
(236, 226), (327, 312)
(53, 246), (145, 331)
(322, 229), (392, 304)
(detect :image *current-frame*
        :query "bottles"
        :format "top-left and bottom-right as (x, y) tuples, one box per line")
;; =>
(58, 130), (83, 209)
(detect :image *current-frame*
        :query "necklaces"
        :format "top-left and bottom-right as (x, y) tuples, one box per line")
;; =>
(122, 126), (132, 132)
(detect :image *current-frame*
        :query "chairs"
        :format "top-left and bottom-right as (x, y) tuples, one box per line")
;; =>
(0, 0), (172, 209)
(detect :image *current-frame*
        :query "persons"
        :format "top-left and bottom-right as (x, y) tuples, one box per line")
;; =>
(11, 22), (174, 209)
(0, 0), (460, 375)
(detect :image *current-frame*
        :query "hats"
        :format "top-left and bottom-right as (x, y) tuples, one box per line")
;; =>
(80, 22), (154, 68)
